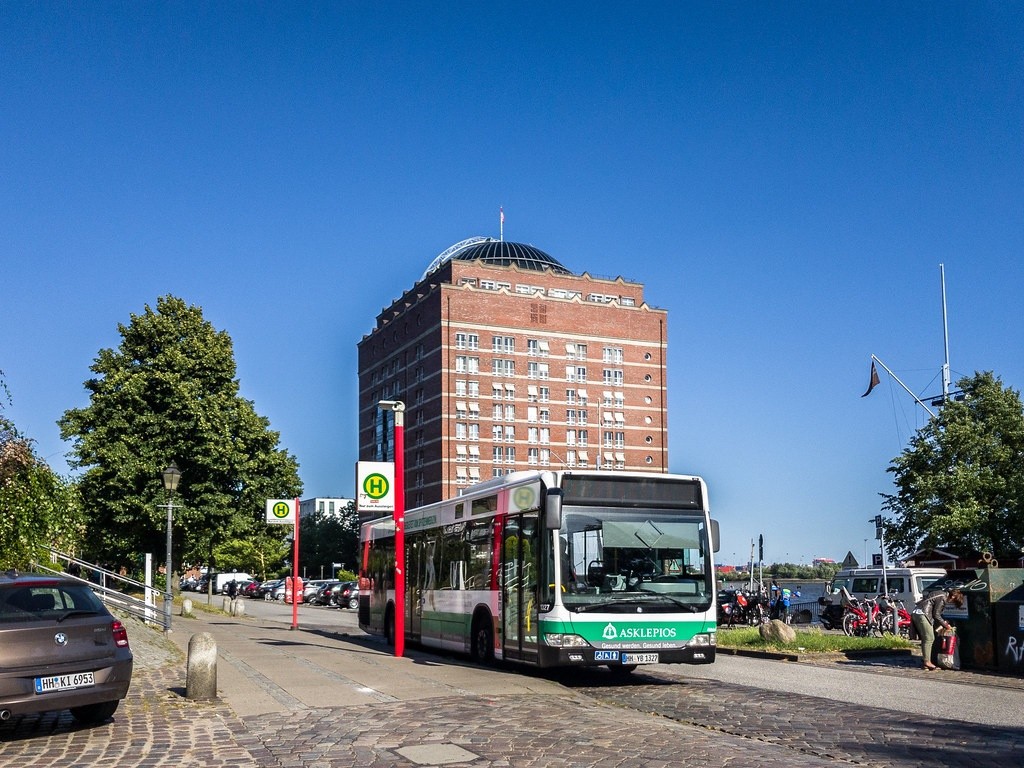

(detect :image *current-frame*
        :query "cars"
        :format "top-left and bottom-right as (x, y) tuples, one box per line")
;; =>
(315, 582), (345, 606)
(180, 580), (216, 593)
(0, 569), (133, 723)
(303, 581), (328, 603)
(222, 581), (279, 598)
(337, 582), (359, 609)
(271, 584), (286, 600)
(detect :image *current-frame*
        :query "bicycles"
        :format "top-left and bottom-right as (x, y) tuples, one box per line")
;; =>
(842, 593), (912, 638)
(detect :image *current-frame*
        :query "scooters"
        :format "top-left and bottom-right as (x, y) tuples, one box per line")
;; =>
(818, 595), (859, 631)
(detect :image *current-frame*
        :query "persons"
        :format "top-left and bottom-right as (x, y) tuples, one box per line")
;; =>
(911, 589), (964, 670)
(550, 536), (577, 592)
(228, 579), (238, 599)
(250, 581), (254, 589)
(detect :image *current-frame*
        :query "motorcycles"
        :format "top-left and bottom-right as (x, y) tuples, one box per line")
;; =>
(717, 580), (802, 625)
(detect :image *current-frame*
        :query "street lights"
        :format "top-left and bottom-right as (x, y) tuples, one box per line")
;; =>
(157, 460), (183, 631)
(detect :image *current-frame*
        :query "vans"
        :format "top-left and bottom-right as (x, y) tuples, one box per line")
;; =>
(216, 573), (257, 595)
(819, 567), (945, 613)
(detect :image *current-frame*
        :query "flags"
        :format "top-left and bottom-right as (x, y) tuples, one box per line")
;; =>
(860, 361), (880, 398)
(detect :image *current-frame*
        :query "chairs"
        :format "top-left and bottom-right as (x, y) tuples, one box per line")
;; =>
(32, 594), (56, 611)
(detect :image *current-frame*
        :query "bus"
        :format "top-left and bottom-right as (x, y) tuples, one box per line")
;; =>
(357, 469), (721, 676)
